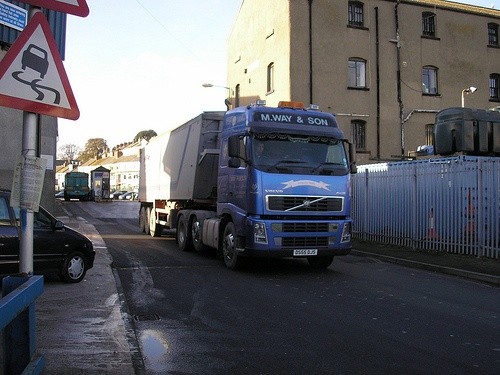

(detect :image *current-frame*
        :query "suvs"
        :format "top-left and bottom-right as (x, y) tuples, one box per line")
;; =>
(0, 189), (96, 283)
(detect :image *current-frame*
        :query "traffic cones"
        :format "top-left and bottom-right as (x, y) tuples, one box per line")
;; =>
(426, 209), (439, 239)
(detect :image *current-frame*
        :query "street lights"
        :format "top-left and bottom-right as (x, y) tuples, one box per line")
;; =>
(203, 83), (231, 112)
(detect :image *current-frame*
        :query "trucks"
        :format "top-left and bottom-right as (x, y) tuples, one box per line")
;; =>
(64, 172), (91, 202)
(138, 100), (356, 270)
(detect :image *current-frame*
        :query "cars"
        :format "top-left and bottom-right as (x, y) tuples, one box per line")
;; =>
(109, 191), (138, 200)
(54, 192), (63, 198)
